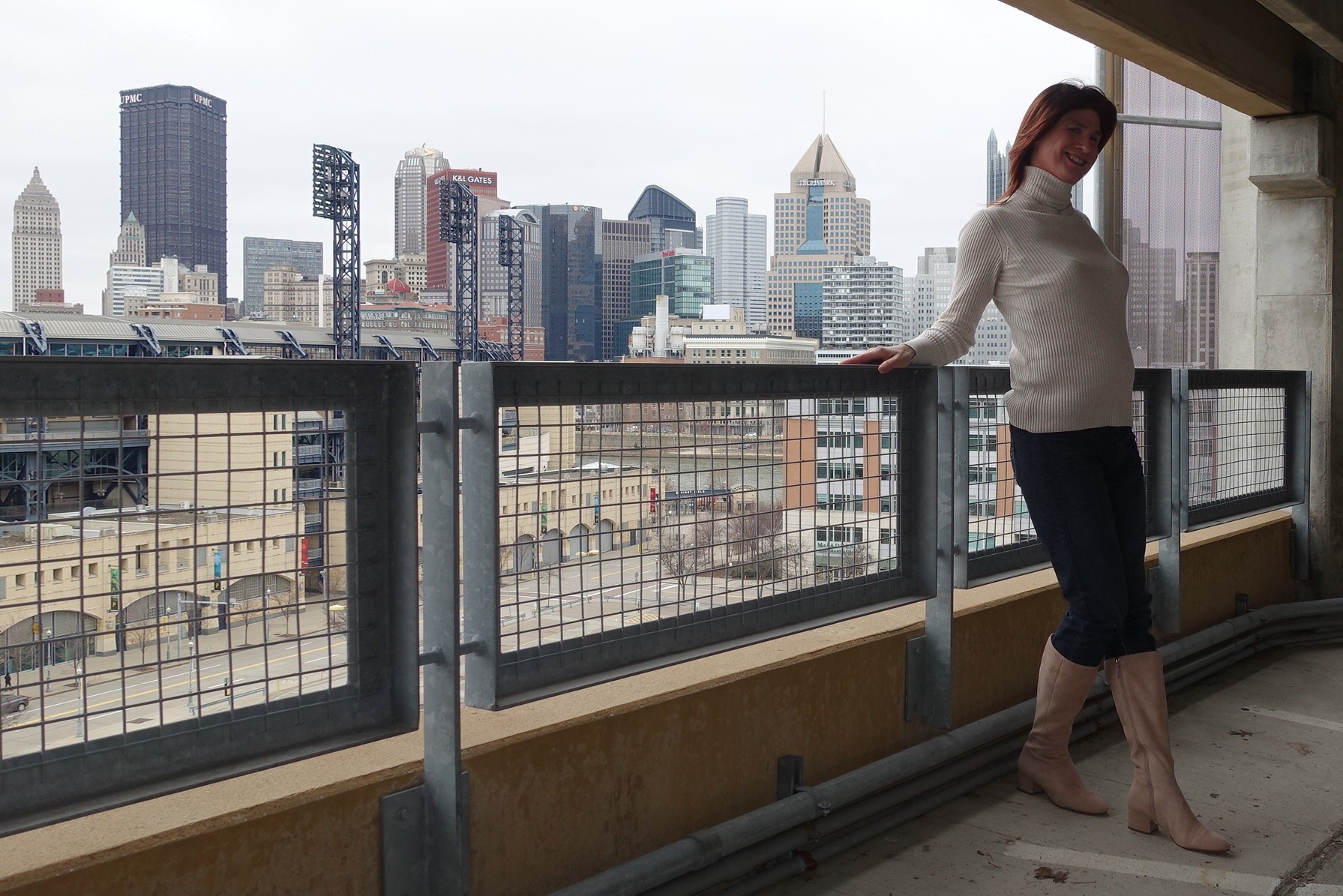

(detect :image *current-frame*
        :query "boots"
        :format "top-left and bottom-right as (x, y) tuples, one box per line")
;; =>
(1104, 651), (1231, 852)
(1016, 633), (1109, 815)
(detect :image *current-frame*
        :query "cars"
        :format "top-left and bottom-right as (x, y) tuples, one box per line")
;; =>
(777, 433), (784, 437)
(657, 428), (669, 434)
(745, 432), (756, 437)
(738, 444), (752, 450)
(1, 694), (30, 714)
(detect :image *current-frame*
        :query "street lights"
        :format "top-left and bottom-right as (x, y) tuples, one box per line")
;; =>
(166, 607), (171, 661)
(45, 628), (53, 692)
(188, 641), (195, 706)
(531, 537), (538, 577)
(76, 668), (84, 737)
(267, 589), (272, 641)
(669, 511), (672, 542)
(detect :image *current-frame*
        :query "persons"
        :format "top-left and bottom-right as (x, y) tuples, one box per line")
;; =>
(840, 75), (1231, 853)
(4, 672), (13, 692)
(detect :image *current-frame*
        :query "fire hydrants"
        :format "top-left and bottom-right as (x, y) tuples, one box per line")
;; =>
(534, 604), (537, 612)
